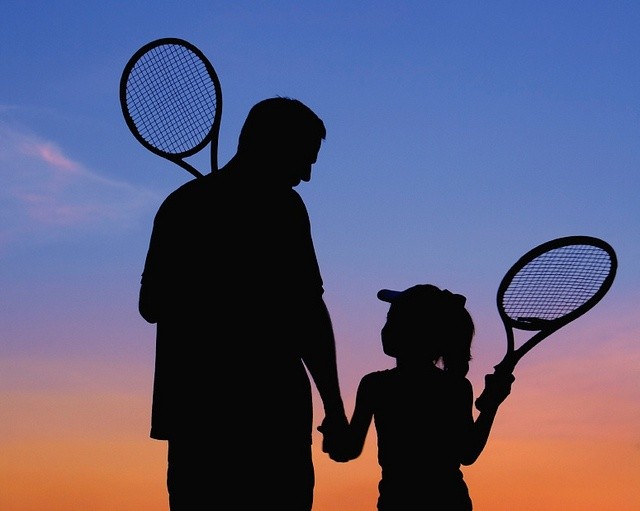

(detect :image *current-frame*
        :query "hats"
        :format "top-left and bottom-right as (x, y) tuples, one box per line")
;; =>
(377, 282), (474, 341)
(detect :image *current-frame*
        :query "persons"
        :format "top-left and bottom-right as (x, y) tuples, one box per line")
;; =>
(138, 93), (349, 511)
(316, 283), (512, 511)
